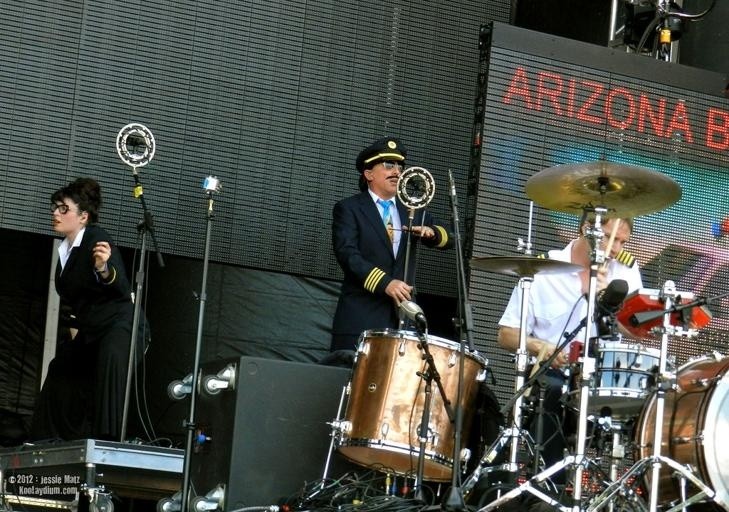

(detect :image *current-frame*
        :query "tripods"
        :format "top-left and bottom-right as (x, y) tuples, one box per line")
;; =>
(460, 277), (559, 505)
(477, 205), (646, 512)
(585, 280), (729, 512)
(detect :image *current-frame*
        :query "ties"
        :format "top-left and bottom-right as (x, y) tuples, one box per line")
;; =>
(376, 198), (394, 251)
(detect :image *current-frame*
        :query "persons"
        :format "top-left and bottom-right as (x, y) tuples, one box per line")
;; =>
(330, 137), (455, 355)
(25, 178), (152, 445)
(498, 216), (644, 484)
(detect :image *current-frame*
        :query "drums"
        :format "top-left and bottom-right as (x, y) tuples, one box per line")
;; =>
(631, 349), (729, 512)
(332, 328), (488, 483)
(568, 341), (673, 420)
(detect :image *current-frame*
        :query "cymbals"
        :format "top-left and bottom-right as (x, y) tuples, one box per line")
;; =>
(468, 255), (587, 276)
(525, 161), (682, 219)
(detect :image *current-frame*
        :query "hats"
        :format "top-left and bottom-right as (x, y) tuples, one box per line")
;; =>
(355, 134), (410, 175)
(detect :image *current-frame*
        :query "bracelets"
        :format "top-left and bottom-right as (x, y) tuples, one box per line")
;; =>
(91, 260), (109, 280)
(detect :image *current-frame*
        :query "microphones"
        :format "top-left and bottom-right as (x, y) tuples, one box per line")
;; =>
(396, 166), (436, 218)
(115, 123), (156, 174)
(202, 174), (221, 217)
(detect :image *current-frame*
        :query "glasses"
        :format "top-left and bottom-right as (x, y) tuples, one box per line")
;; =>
(48, 201), (84, 215)
(382, 158), (407, 172)
